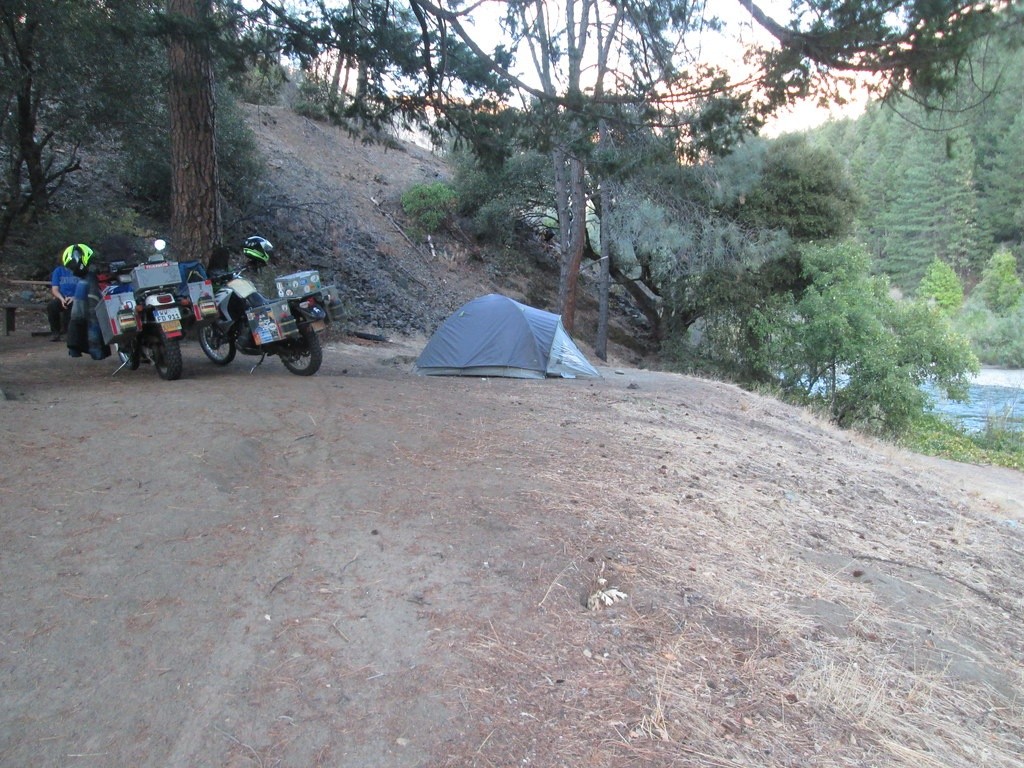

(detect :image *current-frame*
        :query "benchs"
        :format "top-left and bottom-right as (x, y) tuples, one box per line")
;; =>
(0, 303), (48, 336)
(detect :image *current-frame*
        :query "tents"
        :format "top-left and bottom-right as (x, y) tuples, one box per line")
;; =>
(416, 293), (604, 381)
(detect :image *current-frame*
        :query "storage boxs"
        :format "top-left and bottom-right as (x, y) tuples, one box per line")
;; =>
(245, 299), (297, 345)
(177, 280), (217, 323)
(130, 262), (183, 295)
(275, 271), (321, 300)
(317, 286), (345, 324)
(94, 291), (142, 346)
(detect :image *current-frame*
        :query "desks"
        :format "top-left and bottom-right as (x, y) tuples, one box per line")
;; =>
(10, 279), (52, 336)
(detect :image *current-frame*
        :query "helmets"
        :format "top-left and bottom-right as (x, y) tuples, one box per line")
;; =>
(244, 235), (273, 262)
(63, 244), (94, 277)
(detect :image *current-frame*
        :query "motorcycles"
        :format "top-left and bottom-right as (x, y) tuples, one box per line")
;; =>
(186, 244), (331, 377)
(87, 239), (185, 381)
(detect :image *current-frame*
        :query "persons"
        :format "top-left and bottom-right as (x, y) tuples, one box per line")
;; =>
(47, 264), (81, 342)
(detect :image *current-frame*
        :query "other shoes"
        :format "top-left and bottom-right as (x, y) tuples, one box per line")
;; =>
(51, 332), (61, 341)
